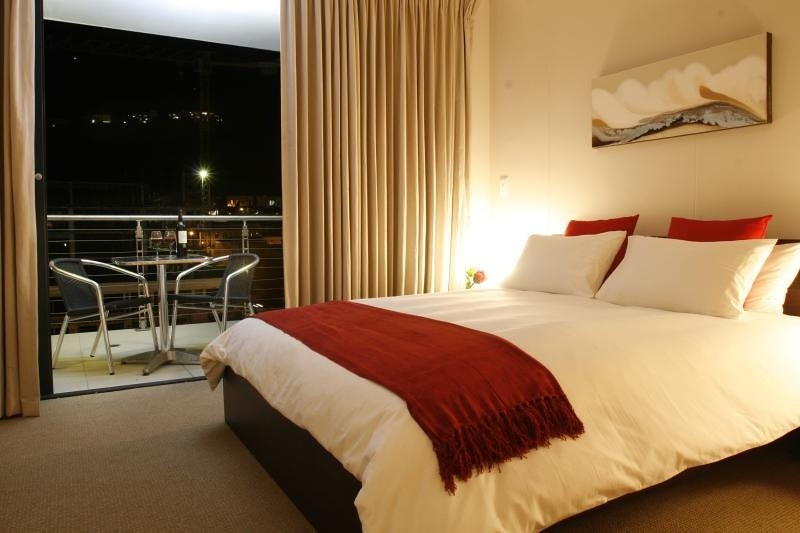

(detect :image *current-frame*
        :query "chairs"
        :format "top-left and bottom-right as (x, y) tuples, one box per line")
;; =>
(170, 252), (259, 349)
(48, 258), (158, 375)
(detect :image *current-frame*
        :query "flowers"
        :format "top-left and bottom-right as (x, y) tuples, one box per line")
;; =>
(465, 267), (487, 290)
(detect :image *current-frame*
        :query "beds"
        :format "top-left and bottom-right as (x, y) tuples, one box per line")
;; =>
(199, 238), (800, 533)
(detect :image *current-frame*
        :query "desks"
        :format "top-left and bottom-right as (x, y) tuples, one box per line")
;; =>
(112, 253), (209, 374)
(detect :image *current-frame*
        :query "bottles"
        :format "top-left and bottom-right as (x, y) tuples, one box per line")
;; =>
(176, 209), (188, 258)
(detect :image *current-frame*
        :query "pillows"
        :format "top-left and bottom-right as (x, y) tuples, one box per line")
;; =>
(502, 231), (627, 298)
(743, 242), (800, 318)
(565, 214), (640, 285)
(596, 234), (777, 319)
(668, 215), (773, 242)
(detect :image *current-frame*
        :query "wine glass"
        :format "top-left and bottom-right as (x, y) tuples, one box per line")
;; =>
(164, 231), (177, 258)
(150, 231), (163, 259)
